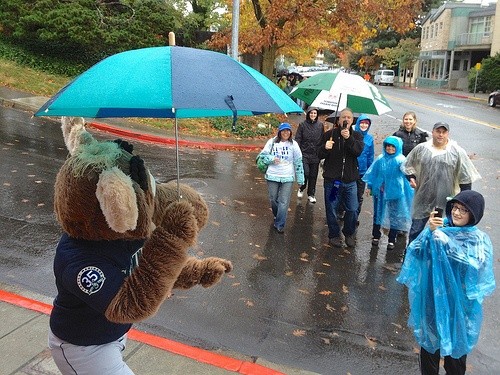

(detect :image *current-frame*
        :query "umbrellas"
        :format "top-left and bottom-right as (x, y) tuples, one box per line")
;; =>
(291, 73), (303, 79)
(326, 111), (361, 126)
(288, 70), (394, 145)
(31, 31), (308, 204)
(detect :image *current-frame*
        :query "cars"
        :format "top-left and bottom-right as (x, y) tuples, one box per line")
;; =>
(287, 64), (351, 74)
(488, 90), (500, 107)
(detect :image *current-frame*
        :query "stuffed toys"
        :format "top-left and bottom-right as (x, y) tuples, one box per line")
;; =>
(48, 115), (234, 375)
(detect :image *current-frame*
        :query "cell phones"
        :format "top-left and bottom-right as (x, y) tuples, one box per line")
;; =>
(434, 207), (444, 217)
(342, 120), (348, 129)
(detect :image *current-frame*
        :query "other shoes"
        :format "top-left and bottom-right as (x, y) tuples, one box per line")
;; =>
(345, 234), (356, 246)
(387, 242), (395, 249)
(297, 188), (306, 198)
(308, 195), (317, 203)
(273, 216), (285, 233)
(330, 237), (341, 247)
(372, 238), (379, 244)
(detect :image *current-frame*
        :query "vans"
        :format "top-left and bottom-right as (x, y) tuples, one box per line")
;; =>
(373, 69), (395, 86)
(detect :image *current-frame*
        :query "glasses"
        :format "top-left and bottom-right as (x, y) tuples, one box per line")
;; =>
(452, 203), (469, 215)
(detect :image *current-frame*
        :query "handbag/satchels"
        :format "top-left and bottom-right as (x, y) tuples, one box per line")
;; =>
(256, 141), (274, 173)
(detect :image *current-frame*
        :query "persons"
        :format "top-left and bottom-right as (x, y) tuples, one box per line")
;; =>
(337, 113), (374, 227)
(364, 71), (371, 82)
(277, 73), (308, 110)
(400, 121), (472, 272)
(392, 112), (429, 160)
(408, 189), (486, 375)
(317, 108), (363, 248)
(294, 107), (324, 203)
(260, 123), (305, 233)
(366, 136), (416, 248)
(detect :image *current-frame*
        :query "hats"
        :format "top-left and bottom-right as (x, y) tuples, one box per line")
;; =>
(433, 122), (450, 131)
(278, 122), (291, 131)
